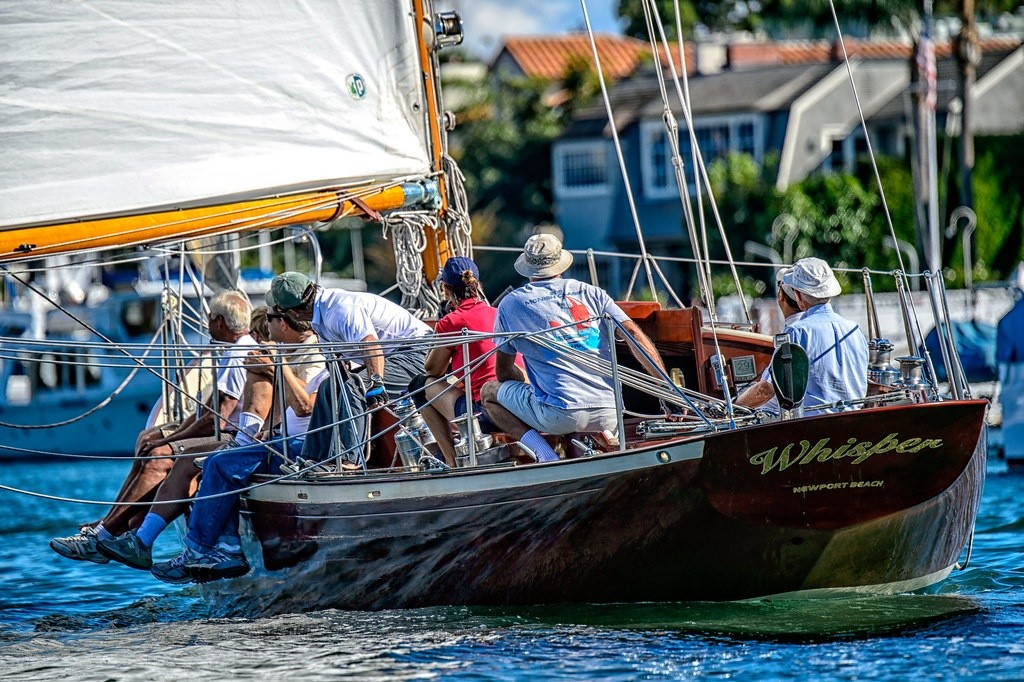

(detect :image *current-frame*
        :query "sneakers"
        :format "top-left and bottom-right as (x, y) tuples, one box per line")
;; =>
(183, 546), (250, 583)
(50, 526), (109, 564)
(193, 437), (242, 469)
(263, 538), (318, 571)
(96, 531), (153, 571)
(152, 547), (199, 585)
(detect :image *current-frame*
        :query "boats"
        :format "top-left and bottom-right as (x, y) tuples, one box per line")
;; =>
(1, 248), (367, 459)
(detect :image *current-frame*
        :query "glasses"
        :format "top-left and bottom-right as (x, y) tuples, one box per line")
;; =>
(265, 313), (283, 322)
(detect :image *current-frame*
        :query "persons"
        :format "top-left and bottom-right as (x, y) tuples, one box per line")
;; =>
(702, 257), (867, 421)
(48, 290), (332, 585)
(265, 270), (435, 476)
(406, 257), (527, 470)
(479, 235), (687, 466)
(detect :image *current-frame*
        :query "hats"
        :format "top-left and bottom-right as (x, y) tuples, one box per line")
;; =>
(266, 271), (313, 308)
(514, 234), (573, 278)
(437, 257), (479, 286)
(783, 257), (841, 298)
(776, 268), (797, 301)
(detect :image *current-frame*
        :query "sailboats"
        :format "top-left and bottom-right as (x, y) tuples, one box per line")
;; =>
(0, 0), (989, 609)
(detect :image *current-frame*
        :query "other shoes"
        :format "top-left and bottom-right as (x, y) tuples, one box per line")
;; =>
(305, 460), (348, 476)
(280, 464), (299, 474)
